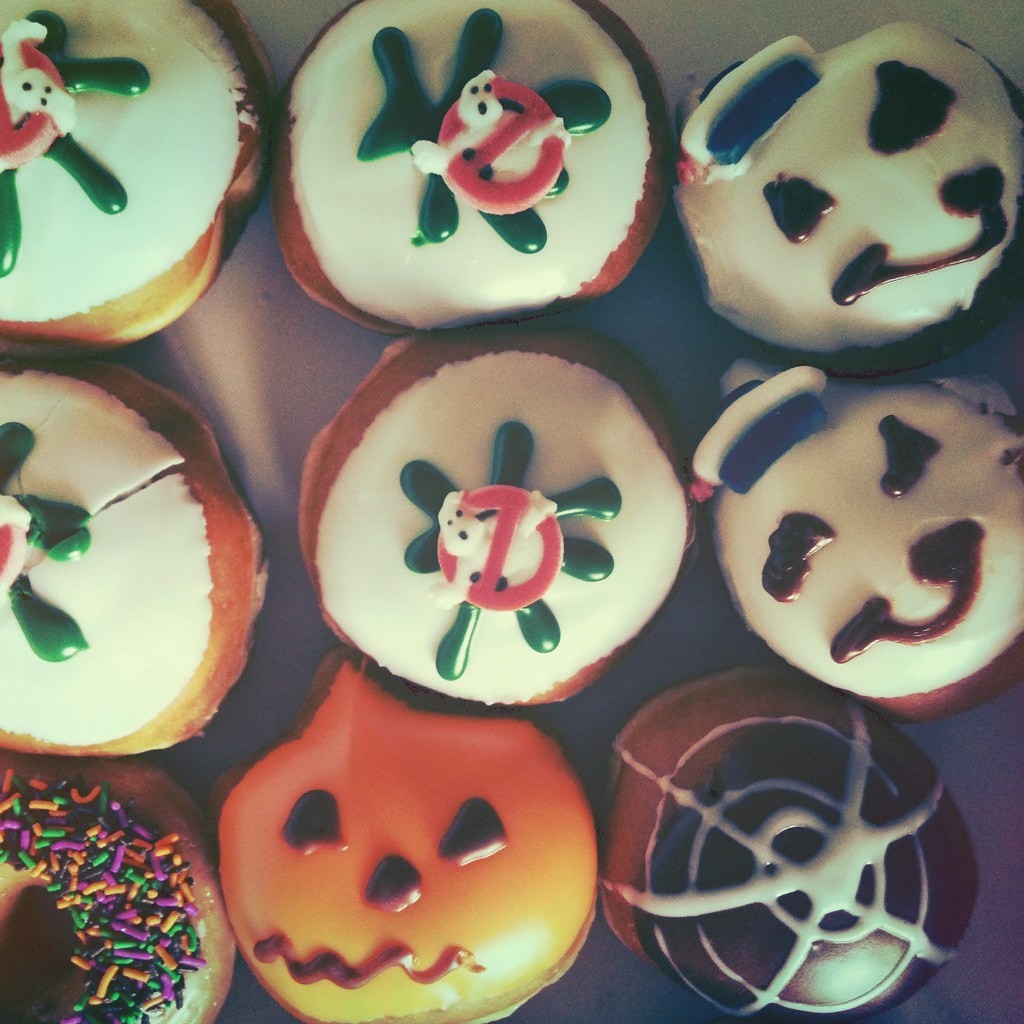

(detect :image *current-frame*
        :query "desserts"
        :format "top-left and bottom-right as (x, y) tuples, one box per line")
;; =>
(0, 0), (1024, 1024)
